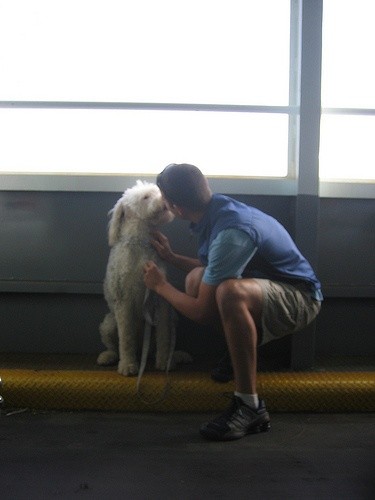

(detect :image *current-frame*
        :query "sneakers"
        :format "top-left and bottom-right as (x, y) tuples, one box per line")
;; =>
(204, 392), (271, 441)
(210, 347), (234, 383)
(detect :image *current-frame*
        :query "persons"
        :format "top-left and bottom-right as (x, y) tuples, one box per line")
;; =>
(142, 163), (322, 443)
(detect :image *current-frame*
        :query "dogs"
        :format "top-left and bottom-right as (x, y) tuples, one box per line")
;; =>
(98, 178), (177, 376)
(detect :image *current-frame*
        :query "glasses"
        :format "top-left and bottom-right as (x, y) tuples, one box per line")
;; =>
(156, 163), (176, 197)
